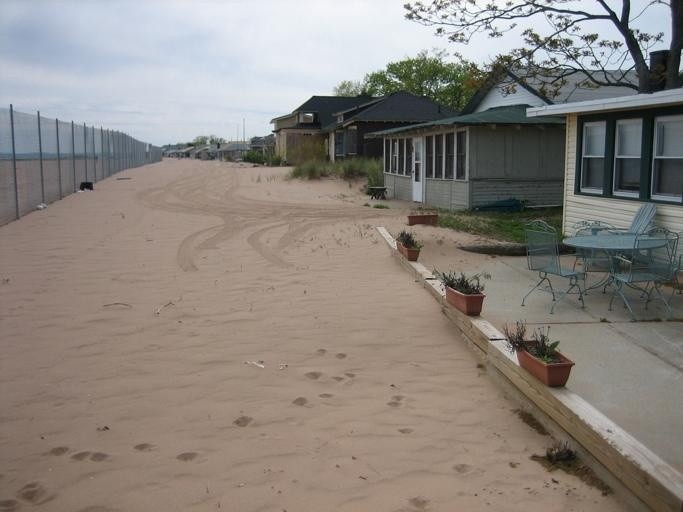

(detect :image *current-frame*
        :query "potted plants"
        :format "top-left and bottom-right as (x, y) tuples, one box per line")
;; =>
(406, 206), (437, 227)
(431, 265), (492, 318)
(394, 228), (424, 263)
(500, 318), (575, 388)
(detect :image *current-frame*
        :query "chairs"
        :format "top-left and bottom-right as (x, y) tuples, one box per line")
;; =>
(520, 202), (682, 323)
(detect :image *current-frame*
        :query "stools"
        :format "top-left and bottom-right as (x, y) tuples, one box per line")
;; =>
(367, 186), (387, 201)
